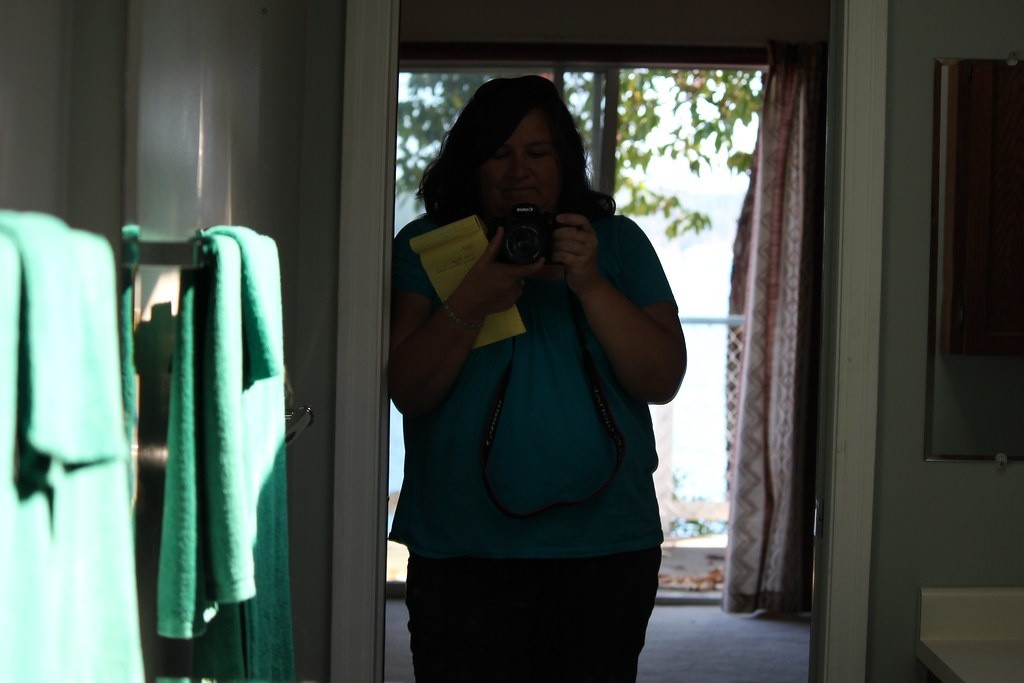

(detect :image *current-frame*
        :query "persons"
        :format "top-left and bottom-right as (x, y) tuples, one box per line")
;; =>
(388, 75), (686, 683)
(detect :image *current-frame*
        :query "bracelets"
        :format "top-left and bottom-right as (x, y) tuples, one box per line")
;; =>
(443, 303), (482, 329)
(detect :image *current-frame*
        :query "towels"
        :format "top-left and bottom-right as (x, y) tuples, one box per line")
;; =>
(155, 223), (296, 683)
(0, 208), (149, 683)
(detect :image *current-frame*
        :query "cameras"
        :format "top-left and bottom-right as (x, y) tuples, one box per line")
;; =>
(483, 201), (572, 265)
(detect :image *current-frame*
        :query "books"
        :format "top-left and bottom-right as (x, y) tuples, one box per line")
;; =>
(408, 214), (527, 347)
